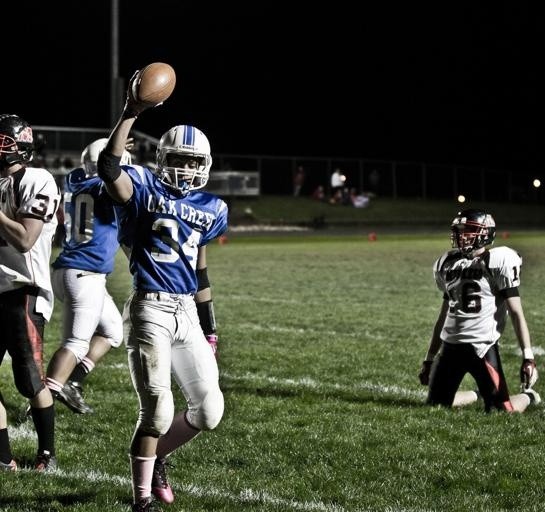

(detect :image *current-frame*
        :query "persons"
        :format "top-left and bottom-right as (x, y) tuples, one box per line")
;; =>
(25, 137), (135, 420)
(289, 163), (378, 210)
(29, 133), (75, 169)
(0, 113), (59, 474)
(418, 208), (542, 415)
(97, 67), (229, 512)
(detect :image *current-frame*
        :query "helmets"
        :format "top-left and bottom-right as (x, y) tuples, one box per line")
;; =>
(81, 138), (133, 178)
(153, 125), (213, 190)
(449, 209), (496, 253)
(0, 113), (36, 168)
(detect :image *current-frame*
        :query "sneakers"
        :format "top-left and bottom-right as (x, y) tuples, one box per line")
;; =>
(59, 381), (91, 413)
(1, 461), (17, 472)
(523, 388), (544, 407)
(152, 458), (175, 502)
(135, 494), (153, 511)
(35, 454), (57, 473)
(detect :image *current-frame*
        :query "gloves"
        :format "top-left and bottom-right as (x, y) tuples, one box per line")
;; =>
(206, 334), (219, 355)
(123, 70), (163, 115)
(520, 357), (539, 390)
(417, 361), (431, 385)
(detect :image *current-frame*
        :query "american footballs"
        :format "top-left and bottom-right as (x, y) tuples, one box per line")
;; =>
(131, 62), (176, 104)
